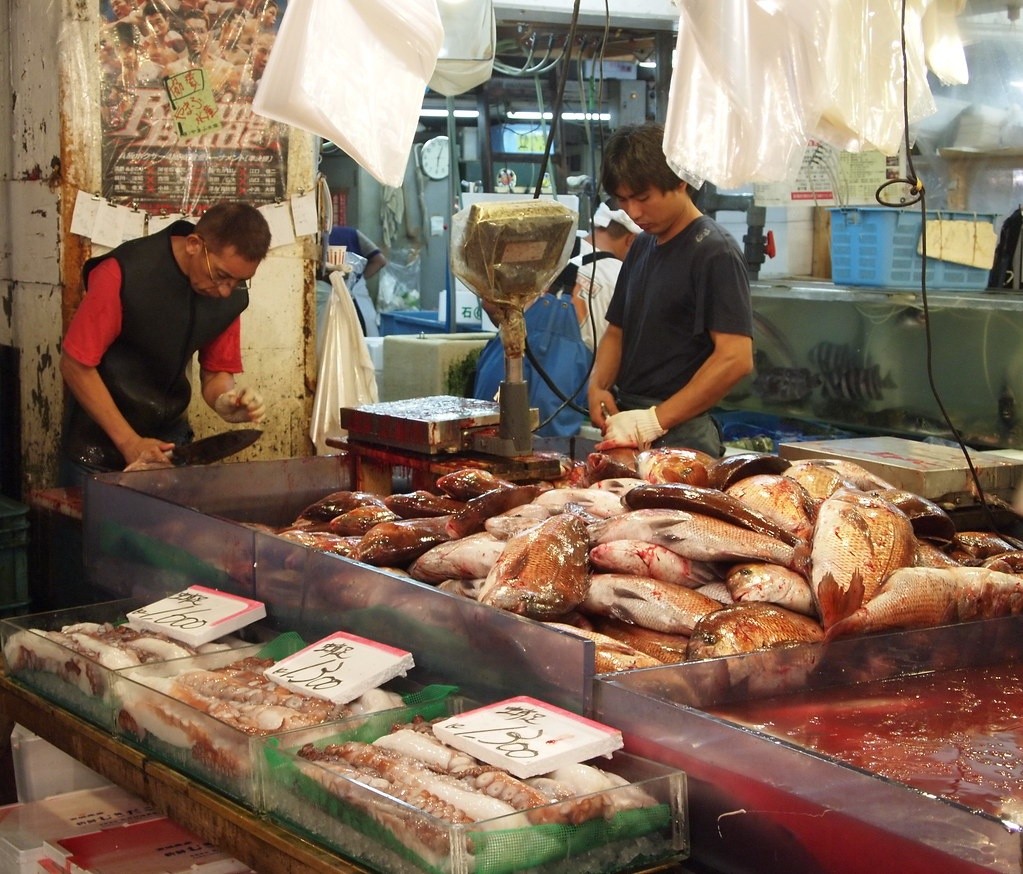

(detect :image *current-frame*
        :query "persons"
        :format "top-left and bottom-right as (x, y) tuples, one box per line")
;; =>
(52, 203), (271, 487)
(586, 121), (759, 458)
(521, 197), (644, 407)
(316, 224), (388, 336)
(988, 203), (1023, 292)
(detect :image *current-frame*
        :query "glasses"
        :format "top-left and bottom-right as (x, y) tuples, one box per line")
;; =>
(198, 235), (252, 292)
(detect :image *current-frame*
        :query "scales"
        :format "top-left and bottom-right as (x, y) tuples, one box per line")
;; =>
(340, 200), (576, 459)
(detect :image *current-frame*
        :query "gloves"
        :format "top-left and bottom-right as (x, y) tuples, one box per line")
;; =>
(594, 405), (669, 452)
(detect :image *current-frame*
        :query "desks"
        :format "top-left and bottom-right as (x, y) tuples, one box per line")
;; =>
(0, 667), (684, 874)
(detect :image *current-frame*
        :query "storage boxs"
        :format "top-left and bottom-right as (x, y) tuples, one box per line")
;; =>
(827, 206), (1002, 293)
(0, 785), (262, 874)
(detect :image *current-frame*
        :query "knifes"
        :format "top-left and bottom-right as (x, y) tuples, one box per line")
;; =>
(161, 429), (264, 465)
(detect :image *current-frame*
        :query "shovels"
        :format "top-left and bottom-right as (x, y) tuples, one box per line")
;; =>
(163, 429), (265, 465)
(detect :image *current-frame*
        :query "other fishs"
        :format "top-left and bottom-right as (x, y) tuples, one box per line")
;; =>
(755, 340), (963, 439)
(281, 442), (1023, 700)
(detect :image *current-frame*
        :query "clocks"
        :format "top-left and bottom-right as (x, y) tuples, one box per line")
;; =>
(419, 136), (450, 180)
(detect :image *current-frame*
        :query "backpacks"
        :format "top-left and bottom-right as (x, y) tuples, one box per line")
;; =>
(474, 236), (619, 438)
